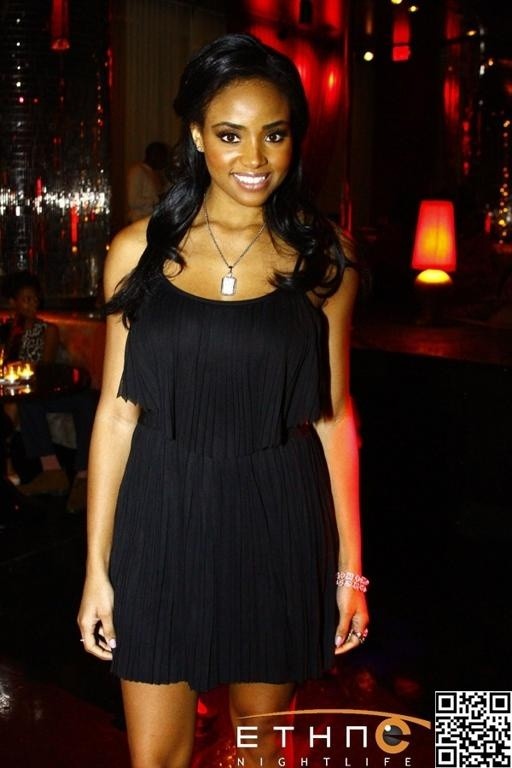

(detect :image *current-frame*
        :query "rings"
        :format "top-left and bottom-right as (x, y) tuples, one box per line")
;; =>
(80, 634), (93, 644)
(351, 629), (369, 643)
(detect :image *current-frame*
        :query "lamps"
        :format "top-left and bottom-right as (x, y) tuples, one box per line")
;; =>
(408, 199), (460, 285)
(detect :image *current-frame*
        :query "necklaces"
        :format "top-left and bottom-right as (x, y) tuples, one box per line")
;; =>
(202, 196), (268, 296)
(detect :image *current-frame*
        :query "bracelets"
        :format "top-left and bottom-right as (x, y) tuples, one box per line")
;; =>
(336, 570), (372, 595)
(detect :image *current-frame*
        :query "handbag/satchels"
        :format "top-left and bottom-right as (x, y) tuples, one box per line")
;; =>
(35, 364), (90, 399)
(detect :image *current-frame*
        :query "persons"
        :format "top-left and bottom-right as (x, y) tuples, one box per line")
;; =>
(0, 272), (60, 426)
(77, 35), (369, 768)
(122, 141), (167, 222)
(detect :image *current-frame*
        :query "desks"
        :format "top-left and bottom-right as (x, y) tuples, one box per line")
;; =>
(1, 362), (71, 463)
